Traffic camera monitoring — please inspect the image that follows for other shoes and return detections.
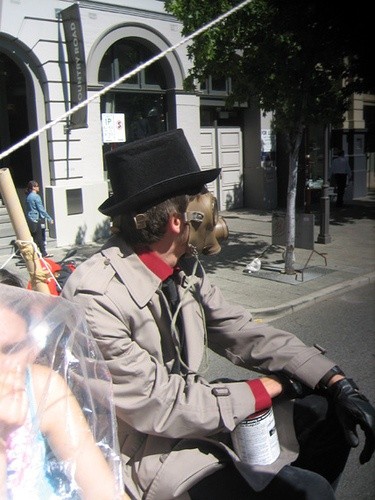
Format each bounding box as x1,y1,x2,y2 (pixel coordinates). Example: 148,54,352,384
45,254,53,257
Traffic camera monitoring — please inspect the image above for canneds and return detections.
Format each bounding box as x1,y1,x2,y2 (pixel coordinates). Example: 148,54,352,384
230,405,280,465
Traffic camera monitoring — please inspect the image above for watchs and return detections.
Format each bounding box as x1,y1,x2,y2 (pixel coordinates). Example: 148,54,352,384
319,365,345,386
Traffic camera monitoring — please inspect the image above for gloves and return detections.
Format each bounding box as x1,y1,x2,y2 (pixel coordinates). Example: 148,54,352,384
274,370,315,399
328,378,375,465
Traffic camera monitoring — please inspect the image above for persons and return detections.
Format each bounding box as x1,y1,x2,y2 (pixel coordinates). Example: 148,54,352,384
47,128,375,500
24,180,54,258
331,150,352,208
0,268,131,499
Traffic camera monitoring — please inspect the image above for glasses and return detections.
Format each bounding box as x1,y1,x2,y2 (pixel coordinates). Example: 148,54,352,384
1,339,29,354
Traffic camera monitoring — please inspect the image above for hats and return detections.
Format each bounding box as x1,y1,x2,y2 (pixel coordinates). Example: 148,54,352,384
98,128,222,218
